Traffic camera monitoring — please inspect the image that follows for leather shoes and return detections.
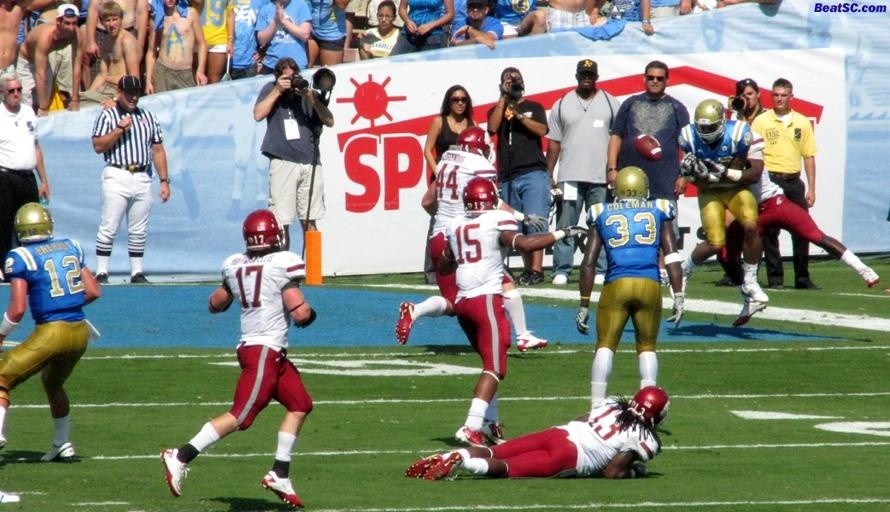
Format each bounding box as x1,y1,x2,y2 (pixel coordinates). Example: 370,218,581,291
95,273,108,283
770,284,784,289
794,278,822,289
130,272,151,283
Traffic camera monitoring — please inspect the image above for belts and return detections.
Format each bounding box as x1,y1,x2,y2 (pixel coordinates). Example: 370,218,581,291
113,164,146,172
769,173,800,180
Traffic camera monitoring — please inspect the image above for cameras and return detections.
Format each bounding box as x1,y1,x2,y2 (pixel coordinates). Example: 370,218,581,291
283,74,312,92
733,97,746,112
507,76,525,92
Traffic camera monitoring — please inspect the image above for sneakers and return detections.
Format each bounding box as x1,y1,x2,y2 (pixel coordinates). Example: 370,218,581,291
664,262,689,298
161,446,191,497
515,270,606,287
732,298,767,327
261,470,306,508
405,418,507,478
516,333,548,352
40,439,77,462
859,267,880,287
741,282,769,303
715,277,735,287
395,301,415,344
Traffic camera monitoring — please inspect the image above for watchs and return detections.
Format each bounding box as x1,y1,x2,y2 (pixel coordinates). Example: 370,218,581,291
159,178,170,182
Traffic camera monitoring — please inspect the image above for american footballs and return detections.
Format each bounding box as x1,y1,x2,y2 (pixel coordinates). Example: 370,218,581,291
634,134,663,160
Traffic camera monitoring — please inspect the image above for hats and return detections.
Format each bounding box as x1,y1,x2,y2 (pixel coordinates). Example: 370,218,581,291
577,59,598,76
467,0,488,6
118,76,142,92
56,3,80,17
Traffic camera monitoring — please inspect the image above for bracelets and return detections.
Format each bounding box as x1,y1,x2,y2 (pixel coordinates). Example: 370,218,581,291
608,167,618,170
116,124,125,129
579,296,589,300
0,312,16,336
553,230,566,240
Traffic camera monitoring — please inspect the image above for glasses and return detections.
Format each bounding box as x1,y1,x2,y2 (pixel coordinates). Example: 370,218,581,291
466,5,484,12
645,75,664,82
449,96,468,104
377,14,393,21
7,86,22,94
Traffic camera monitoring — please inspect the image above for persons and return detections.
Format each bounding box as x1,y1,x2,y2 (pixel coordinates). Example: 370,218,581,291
1,202,100,460
0,75,50,277
395,128,548,353
576,166,684,415
162,209,317,508
253,57,333,254
2,0,353,120
360,0,880,324
438,179,589,447
92,76,171,284
405,385,669,480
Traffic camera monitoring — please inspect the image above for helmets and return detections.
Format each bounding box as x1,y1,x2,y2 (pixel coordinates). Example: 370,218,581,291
456,126,491,159
241,211,281,254
462,177,499,214
615,165,650,200
694,99,725,127
626,386,670,426
13,201,53,244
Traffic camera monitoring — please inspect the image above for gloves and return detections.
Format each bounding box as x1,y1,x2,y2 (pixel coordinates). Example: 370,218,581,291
678,152,728,184
521,212,548,233
575,306,591,335
626,461,649,479
559,224,589,239
666,292,685,330
